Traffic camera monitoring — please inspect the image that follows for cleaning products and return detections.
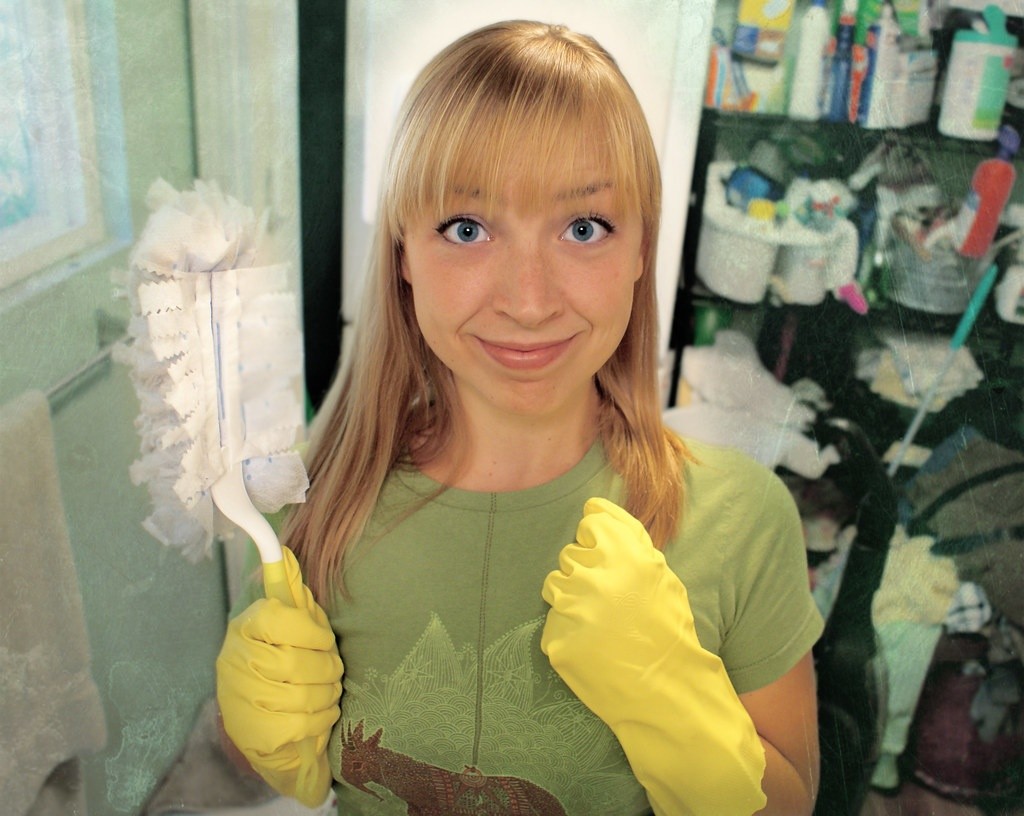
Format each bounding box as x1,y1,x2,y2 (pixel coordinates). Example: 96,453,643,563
789,0,837,120
823,0,900,130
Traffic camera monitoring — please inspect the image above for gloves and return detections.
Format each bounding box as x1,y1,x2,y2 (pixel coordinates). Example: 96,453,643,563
215,546,344,808
540,499,769,814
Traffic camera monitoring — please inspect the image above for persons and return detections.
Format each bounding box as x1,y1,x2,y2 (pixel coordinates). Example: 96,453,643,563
216,19,827,816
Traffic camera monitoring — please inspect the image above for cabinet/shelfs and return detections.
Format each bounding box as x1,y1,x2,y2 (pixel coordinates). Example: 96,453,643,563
658,0,1023,470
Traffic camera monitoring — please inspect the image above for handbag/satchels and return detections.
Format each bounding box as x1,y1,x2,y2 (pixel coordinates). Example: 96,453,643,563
895,423,1024,631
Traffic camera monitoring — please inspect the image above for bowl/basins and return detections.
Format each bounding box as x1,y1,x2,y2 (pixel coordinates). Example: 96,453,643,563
882,243,973,314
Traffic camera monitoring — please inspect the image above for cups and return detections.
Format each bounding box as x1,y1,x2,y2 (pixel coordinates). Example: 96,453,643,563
937,4,1020,143
